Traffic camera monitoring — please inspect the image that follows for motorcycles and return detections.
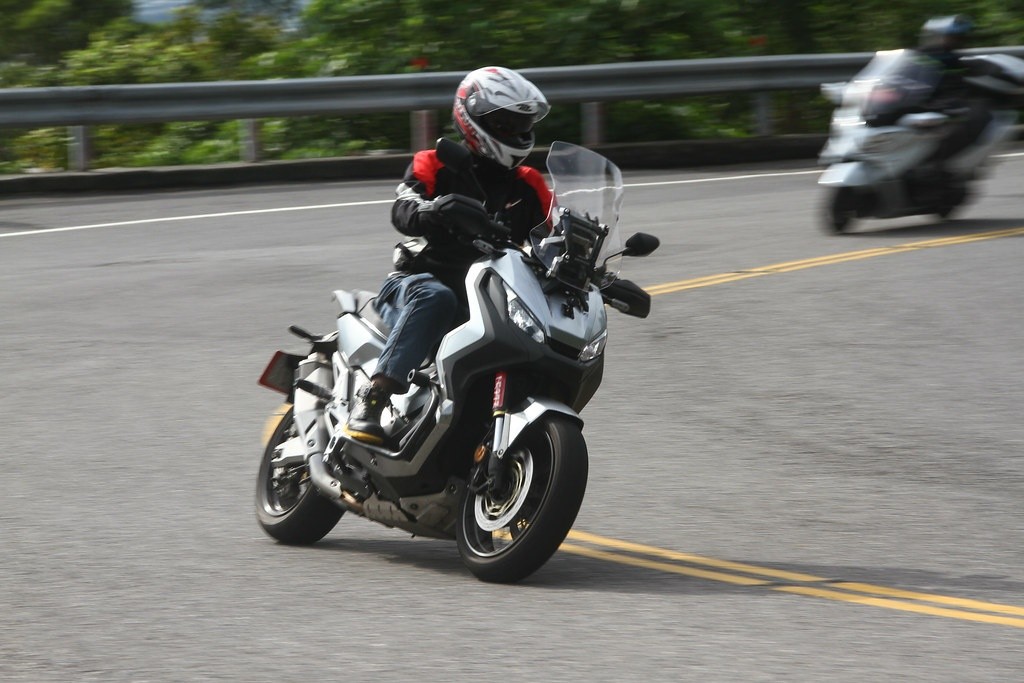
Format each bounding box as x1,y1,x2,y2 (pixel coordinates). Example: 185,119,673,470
254,142,664,588
808,46,1023,237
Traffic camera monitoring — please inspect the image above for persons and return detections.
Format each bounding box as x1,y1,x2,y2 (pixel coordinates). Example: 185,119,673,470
342,66,571,444
869,13,993,214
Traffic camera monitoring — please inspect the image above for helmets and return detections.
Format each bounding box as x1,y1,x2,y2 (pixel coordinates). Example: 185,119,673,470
453,66,550,169
924,15,967,49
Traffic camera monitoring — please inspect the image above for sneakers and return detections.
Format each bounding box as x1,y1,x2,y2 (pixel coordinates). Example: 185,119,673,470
343,387,390,444
